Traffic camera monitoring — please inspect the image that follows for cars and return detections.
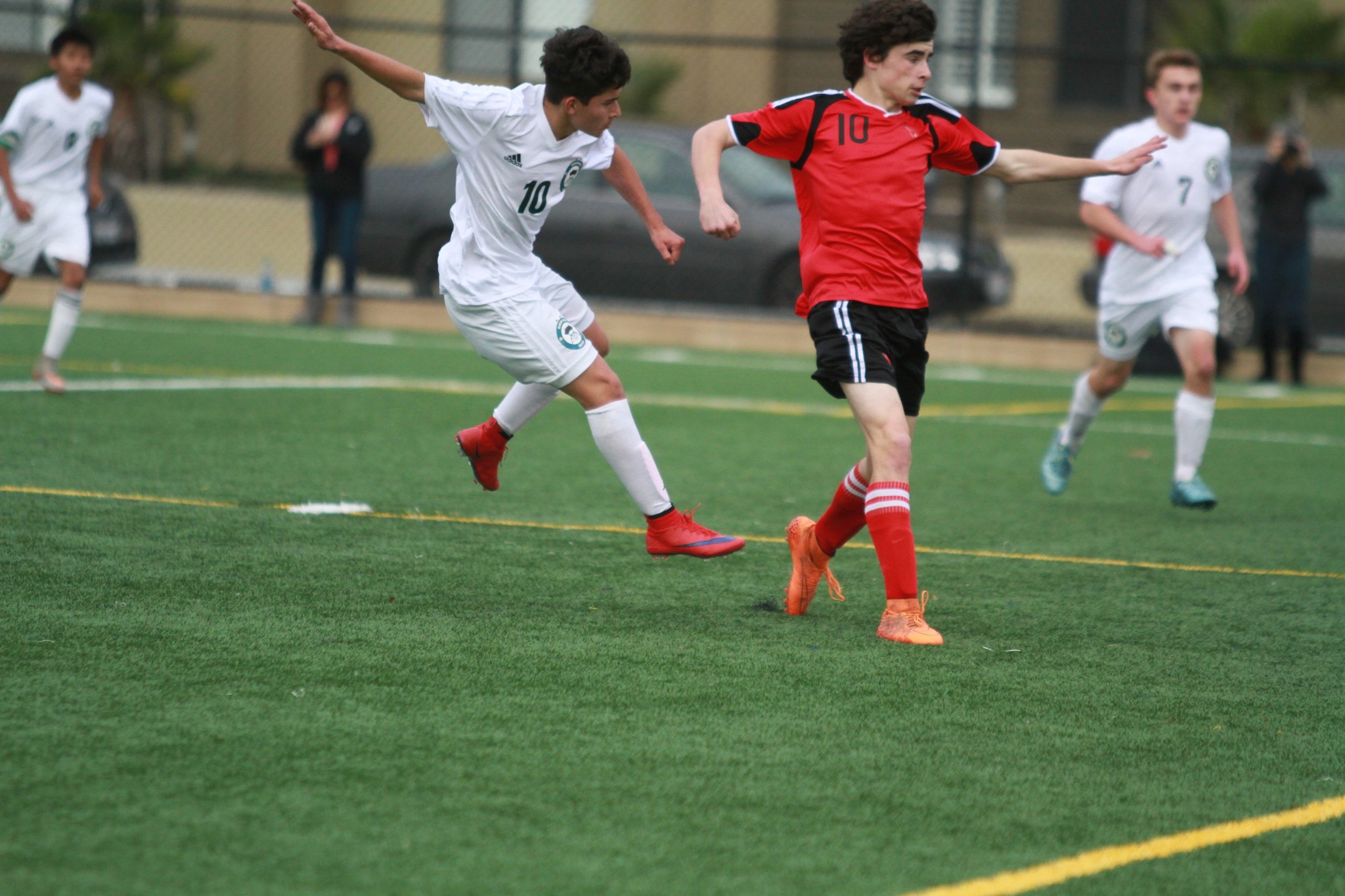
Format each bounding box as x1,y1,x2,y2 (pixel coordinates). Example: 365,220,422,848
31,174,139,280
353,117,1015,322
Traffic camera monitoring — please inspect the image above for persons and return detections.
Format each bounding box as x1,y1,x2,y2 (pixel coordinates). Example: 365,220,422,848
1039,48,1252,509
690,0,1168,646
289,0,747,560
1248,122,1329,386
0,27,114,393
289,66,373,328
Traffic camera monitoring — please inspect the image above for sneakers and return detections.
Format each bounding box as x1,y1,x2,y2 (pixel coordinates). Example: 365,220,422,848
453,414,514,492
31,351,66,393
643,502,746,562
1039,425,1082,496
784,516,845,616
876,591,944,645
1169,472,1218,509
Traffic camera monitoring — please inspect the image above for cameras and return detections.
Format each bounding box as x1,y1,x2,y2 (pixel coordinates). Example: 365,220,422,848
1283,145,1298,156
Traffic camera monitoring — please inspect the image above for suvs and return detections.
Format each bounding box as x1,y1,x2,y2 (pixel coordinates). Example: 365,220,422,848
1074,142,1345,380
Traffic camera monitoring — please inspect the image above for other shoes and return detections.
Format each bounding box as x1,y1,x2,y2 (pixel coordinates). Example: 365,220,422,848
335,291,357,326
292,291,326,325
